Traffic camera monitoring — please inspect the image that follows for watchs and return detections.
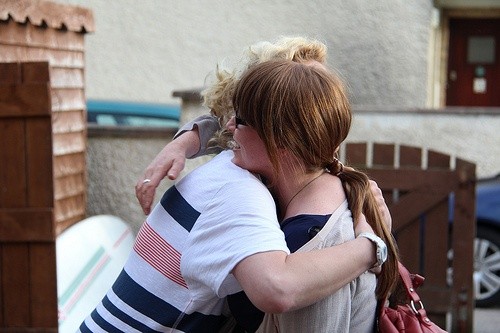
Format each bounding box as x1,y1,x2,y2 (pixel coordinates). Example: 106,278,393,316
355,232,387,268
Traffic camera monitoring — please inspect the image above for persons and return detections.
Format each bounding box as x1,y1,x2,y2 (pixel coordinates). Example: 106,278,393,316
72,35,394,333
134,61,387,333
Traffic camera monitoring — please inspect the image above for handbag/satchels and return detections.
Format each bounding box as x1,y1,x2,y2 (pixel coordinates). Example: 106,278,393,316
378,261,448,333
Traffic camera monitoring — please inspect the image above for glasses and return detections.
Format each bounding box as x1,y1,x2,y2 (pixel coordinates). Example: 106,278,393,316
236,111,249,129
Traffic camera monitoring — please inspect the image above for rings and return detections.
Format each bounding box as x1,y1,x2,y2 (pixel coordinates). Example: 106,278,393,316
142,179,150,183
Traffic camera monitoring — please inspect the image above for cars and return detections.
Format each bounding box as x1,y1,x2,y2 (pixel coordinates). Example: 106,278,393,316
391,172,500,306
85,98,181,128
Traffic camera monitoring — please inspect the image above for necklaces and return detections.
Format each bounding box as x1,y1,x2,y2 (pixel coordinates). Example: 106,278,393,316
284,169,327,221
